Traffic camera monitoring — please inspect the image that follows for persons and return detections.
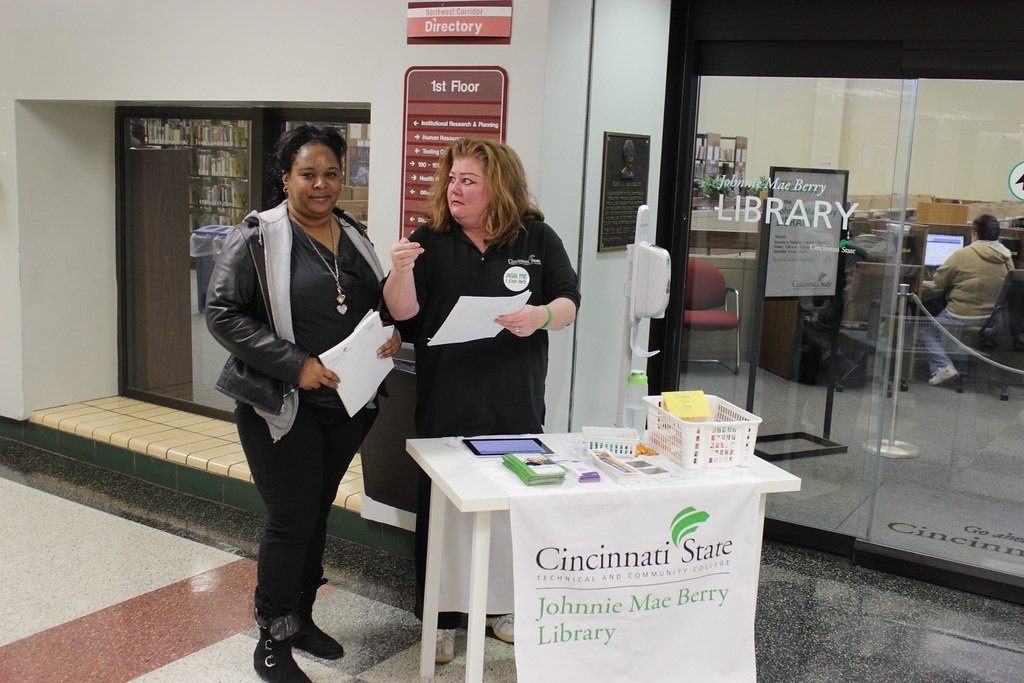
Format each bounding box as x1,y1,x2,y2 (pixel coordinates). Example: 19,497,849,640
801,217,903,386
737,170,743,187
204,123,402,683
378,138,580,662
919,214,1016,386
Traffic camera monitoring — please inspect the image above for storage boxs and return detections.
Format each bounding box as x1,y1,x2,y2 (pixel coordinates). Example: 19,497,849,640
641,393,764,470
917,202,968,225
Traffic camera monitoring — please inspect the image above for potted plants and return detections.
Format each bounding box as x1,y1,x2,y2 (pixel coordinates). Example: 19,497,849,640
696,173,732,199
756,177,768,200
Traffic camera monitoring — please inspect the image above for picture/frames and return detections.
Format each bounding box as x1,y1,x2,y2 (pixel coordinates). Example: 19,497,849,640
597,131,651,251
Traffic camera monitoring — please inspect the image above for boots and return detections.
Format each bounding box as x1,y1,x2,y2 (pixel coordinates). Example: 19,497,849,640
292,566,344,660
254,585,312,682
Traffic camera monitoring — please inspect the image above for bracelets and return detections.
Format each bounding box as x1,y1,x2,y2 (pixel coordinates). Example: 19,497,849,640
540,305,553,329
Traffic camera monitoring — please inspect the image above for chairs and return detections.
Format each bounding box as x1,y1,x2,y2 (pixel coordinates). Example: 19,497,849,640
814,261,923,397
937,268,1024,401
682,262,741,374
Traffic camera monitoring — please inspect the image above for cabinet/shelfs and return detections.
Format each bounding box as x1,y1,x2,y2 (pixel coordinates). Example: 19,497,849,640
130,118,247,267
693,133,747,195
680,210,759,358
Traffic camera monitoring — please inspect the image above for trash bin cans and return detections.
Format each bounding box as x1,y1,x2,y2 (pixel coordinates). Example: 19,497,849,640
359,344,421,514
192,225,235,314
794,297,843,386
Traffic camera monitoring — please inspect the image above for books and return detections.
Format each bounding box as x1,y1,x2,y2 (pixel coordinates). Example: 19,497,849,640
189,206,249,236
197,146,249,177
695,145,706,159
719,148,733,161
190,177,250,207
142,118,249,148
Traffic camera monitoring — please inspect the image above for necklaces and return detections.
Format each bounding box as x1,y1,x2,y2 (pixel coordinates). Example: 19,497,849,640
288,212,348,316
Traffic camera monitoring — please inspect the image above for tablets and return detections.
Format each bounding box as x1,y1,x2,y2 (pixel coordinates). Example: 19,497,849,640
462,437,557,457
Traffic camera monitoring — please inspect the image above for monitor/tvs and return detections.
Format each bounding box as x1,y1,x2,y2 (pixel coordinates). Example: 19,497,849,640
1002,237,1021,265
924,233,966,267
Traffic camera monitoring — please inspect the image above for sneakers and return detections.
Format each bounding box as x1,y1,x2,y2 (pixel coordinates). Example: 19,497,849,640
929,365,957,385
435,629,456,662
486,615,513,641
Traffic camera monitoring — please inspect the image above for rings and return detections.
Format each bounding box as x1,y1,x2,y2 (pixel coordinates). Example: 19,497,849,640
516,327,520,333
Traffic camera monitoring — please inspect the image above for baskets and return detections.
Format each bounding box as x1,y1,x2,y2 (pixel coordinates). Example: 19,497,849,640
643,395,763,469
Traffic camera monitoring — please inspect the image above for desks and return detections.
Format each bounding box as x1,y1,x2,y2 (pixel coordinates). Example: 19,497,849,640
405,437,802,683
